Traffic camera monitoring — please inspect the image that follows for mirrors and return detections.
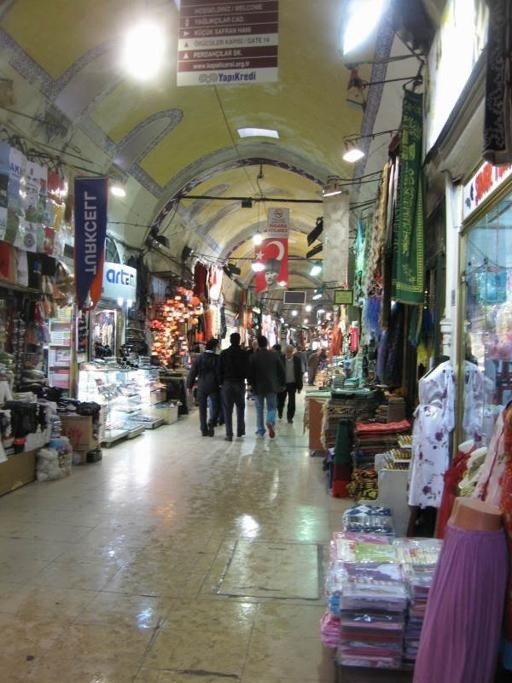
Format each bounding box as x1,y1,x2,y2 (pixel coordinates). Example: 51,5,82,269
453,173,512,457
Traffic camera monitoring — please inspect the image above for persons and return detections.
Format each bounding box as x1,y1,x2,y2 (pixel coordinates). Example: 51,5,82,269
257,258,287,304
213,338,227,428
277,343,302,424
241,334,328,403
214,331,249,443
184,336,222,438
245,334,287,439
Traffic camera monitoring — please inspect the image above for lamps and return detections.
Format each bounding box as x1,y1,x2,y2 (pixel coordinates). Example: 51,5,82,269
342,129,403,163
59,162,127,198
226,201,344,302
322,171,384,198
346,70,423,105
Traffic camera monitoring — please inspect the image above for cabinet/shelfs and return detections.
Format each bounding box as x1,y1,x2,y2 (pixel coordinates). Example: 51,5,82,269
78,366,168,448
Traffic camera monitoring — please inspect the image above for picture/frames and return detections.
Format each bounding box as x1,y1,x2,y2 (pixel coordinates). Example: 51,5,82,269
461,158,512,225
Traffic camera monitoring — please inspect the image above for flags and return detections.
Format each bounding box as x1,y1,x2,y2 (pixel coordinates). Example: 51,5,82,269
254,237,288,304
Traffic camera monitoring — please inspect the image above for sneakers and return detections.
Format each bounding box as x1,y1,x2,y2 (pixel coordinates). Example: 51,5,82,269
277,409,283,420
255,431,264,438
286,418,294,424
224,435,232,441
308,381,317,386
237,432,243,437
266,422,275,439
202,419,224,437
